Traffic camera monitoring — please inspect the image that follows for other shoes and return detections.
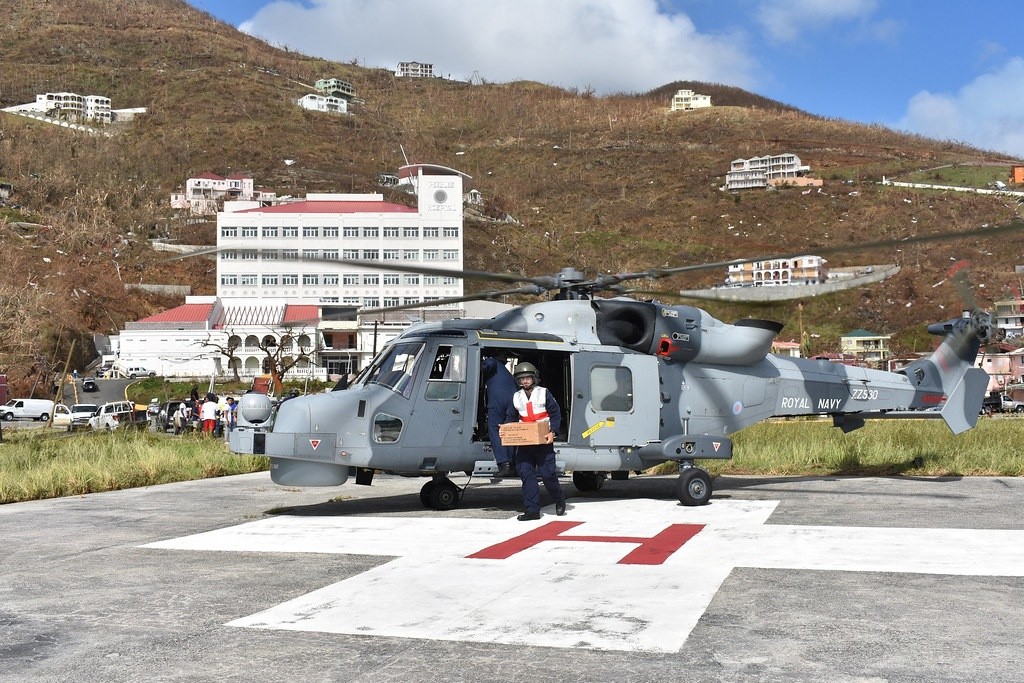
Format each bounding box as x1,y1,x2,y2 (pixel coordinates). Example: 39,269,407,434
493,462,512,476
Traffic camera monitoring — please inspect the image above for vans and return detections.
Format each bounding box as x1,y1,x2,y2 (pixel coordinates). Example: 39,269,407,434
99,364,112,377
0,399,54,423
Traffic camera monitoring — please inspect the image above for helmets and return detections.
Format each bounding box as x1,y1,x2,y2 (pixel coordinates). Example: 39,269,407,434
513,362,541,390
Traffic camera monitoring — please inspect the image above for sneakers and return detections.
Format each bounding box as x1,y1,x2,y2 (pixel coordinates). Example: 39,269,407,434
517,511,540,521
556,501,566,516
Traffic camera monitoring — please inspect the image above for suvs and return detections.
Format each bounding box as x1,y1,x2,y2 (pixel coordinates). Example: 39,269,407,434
126,366,157,381
157,399,190,433
53,403,98,433
149,398,160,415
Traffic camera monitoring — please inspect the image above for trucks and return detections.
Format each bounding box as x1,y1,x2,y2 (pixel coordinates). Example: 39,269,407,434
88,400,148,435
980,392,1024,415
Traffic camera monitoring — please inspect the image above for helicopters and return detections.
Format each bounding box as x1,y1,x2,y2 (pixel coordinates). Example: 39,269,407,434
159,220,1024,514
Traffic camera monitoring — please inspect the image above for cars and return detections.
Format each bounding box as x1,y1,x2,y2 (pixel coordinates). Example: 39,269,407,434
81,376,95,387
83,381,96,393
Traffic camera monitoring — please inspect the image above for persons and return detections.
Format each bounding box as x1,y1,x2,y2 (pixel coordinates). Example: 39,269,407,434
172,392,238,440
497,361,565,521
479,356,520,479
275,387,299,412
73,368,78,378
191,384,199,402
